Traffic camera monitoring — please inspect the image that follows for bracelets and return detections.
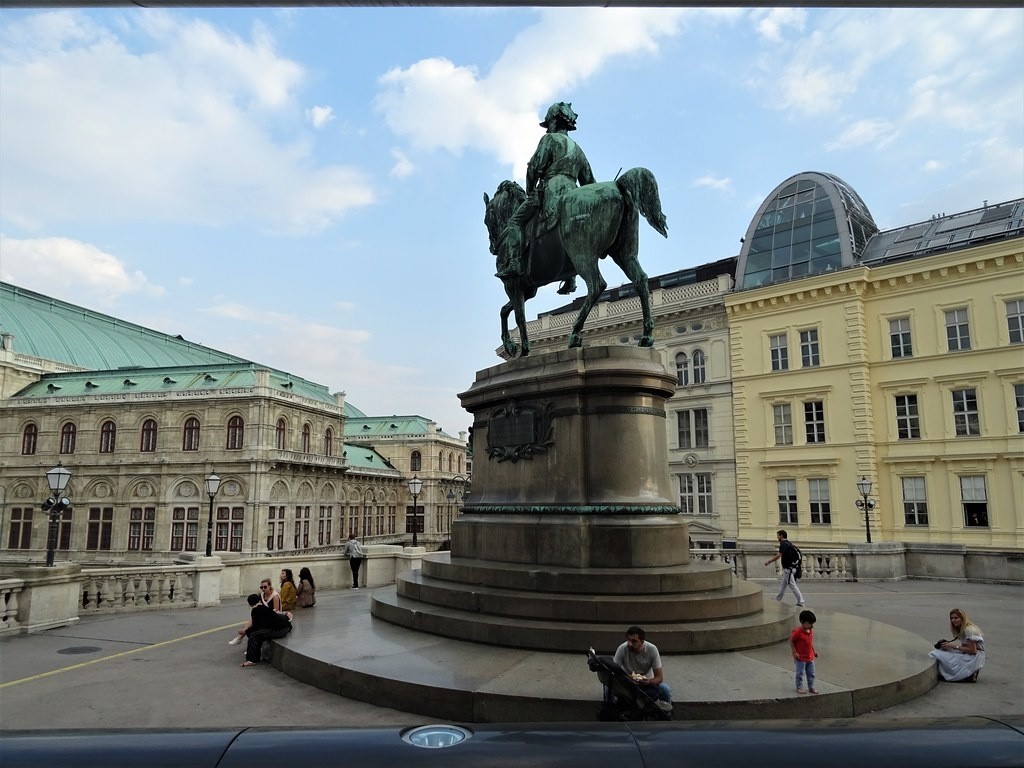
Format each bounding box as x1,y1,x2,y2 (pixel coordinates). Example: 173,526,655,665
956,645,958,649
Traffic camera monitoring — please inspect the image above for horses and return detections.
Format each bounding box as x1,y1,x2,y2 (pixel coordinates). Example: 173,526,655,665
483,166,669,359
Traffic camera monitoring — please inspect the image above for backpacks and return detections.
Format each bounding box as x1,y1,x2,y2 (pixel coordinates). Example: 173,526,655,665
350,541,363,558
786,541,802,568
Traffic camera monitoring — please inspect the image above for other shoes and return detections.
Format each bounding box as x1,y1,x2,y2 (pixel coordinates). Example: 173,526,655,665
350,586,358,590
240,661,258,667
795,601,804,607
809,688,818,694
796,688,807,694
971,669,979,683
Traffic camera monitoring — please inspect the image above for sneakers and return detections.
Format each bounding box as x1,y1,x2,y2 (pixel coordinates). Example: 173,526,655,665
228,638,242,646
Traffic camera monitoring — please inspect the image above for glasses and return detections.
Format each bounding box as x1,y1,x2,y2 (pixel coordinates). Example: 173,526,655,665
260,586,268,590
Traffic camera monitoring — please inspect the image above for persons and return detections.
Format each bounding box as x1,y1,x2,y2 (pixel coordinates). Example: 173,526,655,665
228,578,280,646
790,609,818,694
343,535,363,590
237,593,287,666
495,101,596,292
602,627,671,721
764,530,805,607
295,567,317,607
928,608,985,682
278,568,298,610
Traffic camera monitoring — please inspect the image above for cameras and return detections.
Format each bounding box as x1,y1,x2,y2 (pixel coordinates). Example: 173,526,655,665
934,639,947,651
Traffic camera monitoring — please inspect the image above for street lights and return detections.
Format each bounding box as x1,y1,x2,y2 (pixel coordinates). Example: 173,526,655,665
854,475,876,544
406,474,423,547
204,468,223,557
41,460,73,566
